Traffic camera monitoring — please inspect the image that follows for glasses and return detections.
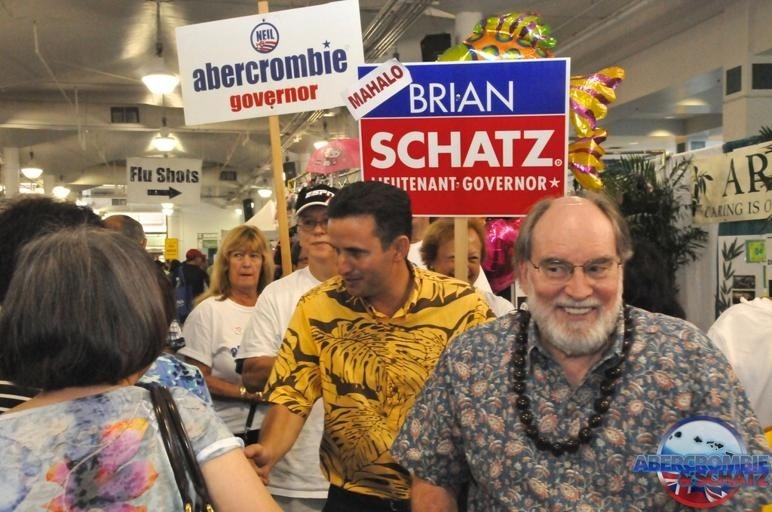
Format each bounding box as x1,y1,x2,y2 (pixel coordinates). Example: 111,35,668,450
527,259,620,279
297,217,329,232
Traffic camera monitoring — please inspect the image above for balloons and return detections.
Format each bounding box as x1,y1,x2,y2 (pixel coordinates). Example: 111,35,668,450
433,13,626,137
568,129,608,195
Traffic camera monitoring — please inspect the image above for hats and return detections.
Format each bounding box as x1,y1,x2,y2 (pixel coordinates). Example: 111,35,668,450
295,184,338,216
186,248,208,257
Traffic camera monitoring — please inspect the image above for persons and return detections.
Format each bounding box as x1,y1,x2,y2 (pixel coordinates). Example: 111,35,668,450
0,179,771,511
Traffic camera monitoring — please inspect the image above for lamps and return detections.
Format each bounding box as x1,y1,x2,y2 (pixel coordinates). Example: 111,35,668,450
235,123,330,224
142,0,180,97
53,174,99,214
20,130,43,179
154,97,176,152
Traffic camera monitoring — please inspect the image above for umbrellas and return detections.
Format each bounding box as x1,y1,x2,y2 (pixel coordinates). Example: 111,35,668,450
303,137,361,174
